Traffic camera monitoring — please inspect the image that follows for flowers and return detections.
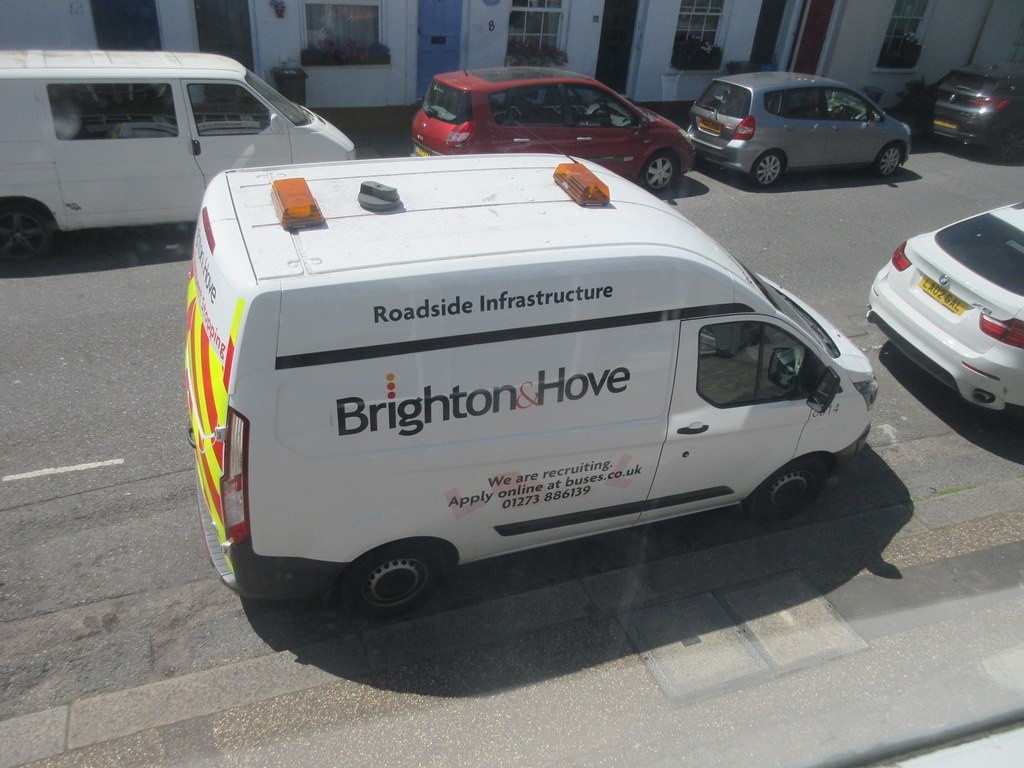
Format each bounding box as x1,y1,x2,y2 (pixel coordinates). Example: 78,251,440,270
301,37,389,62
270,0,284,9
506,39,568,68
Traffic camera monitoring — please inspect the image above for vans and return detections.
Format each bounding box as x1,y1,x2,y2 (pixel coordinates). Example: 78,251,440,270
0,49,358,269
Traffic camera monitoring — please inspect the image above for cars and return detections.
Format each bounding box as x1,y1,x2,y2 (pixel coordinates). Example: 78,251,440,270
863,201,1024,417
911,60,1024,166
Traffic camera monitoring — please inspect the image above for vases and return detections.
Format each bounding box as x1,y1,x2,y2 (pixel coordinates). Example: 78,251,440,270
670,36,720,66
276,8,284,18
684,54,721,69
303,56,390,65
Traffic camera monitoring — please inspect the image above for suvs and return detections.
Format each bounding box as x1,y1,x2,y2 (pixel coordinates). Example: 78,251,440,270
686,70,913,190
409,64,696,195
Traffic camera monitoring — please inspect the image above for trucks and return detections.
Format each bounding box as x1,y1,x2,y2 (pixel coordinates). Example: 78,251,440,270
183,153,880,624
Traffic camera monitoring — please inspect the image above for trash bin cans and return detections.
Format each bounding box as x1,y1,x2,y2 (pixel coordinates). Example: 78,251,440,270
272,68,310,106
726,61,762,76
